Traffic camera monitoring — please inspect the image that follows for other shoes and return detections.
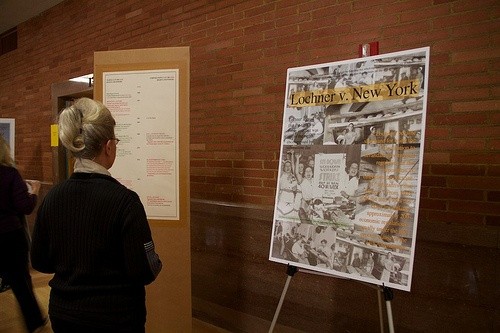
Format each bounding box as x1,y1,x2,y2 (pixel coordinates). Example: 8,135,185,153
31,318,48,333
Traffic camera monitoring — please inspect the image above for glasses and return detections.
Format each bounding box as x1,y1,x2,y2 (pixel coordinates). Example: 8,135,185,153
105,137,120,145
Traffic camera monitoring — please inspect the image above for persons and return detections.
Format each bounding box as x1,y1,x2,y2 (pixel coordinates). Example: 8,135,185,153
272,54,424,282
0,134,50,333
30,97,163,333
